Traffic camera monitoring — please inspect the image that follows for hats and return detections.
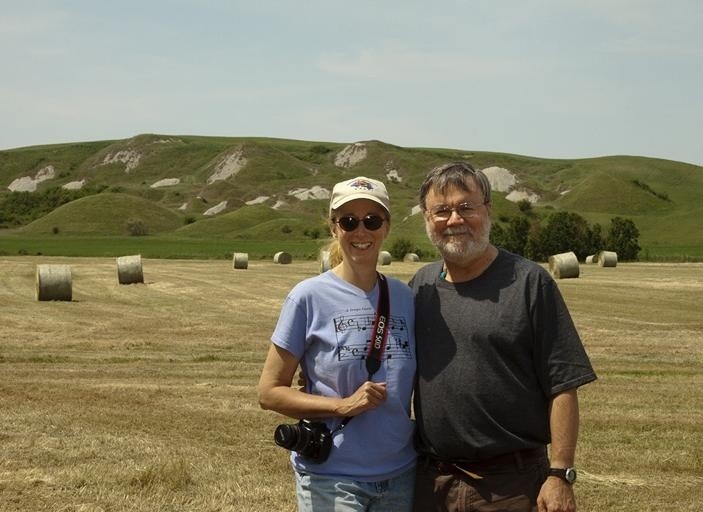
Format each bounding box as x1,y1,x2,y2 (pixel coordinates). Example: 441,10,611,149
329,176,392,220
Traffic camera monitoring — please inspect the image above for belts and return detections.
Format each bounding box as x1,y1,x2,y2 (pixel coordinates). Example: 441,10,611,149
423,445,546,480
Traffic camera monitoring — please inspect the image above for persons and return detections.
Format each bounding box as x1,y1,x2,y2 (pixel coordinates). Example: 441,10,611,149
257,177,420,512
298,163,598,512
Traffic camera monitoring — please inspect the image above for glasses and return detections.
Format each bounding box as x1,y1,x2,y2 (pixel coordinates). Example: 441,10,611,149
334,213,387,230
425,201,484,221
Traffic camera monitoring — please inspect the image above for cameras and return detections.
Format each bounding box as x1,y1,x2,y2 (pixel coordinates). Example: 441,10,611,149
274,419,332,464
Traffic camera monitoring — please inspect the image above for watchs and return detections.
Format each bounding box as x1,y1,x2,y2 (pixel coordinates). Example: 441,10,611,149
547,467,577,485
